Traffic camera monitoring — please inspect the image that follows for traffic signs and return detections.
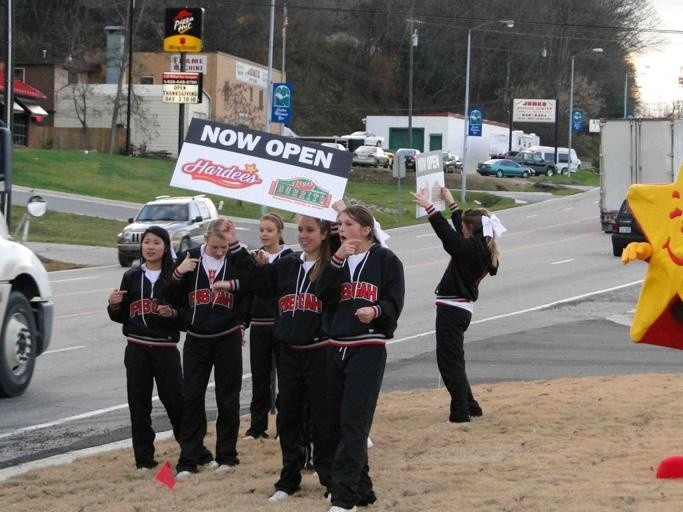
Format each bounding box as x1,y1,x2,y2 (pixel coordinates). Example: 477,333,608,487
161,71,199,104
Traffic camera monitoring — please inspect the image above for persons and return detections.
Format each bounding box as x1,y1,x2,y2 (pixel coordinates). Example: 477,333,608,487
212,216,332,499
323,216,349,285
409,186,506,423
154,215,256,479
107,226,218,474
244,215,301,440
315,206,405,511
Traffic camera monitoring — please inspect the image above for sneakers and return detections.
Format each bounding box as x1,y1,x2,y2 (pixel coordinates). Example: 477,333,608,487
268,490,304,503
367,436,374,449
137,467,147,472
321,492,332,501
242,435,254,441
197,461,219,471
176,471,190,479
328,506,356,512
216,465,234,474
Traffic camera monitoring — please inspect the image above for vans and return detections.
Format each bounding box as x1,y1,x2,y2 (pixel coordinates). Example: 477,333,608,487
0,121,54,397
523,146,577,175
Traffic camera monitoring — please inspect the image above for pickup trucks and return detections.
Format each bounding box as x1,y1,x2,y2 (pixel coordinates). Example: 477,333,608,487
490,151,555,177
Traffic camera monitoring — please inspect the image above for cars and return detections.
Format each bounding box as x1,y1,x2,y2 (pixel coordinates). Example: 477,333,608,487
475,158,531,178
347,130,461,176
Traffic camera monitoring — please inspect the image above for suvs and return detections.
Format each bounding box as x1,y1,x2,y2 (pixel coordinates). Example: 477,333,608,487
116,194,219,268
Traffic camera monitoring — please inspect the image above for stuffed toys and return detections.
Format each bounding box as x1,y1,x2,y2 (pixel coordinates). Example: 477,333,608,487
620,160,683,478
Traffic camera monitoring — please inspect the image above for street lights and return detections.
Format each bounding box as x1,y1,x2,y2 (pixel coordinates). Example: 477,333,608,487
567,46,603,178
460,19,514,206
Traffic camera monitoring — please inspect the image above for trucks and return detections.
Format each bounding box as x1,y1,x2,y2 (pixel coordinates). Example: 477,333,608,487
599,118,682,256
